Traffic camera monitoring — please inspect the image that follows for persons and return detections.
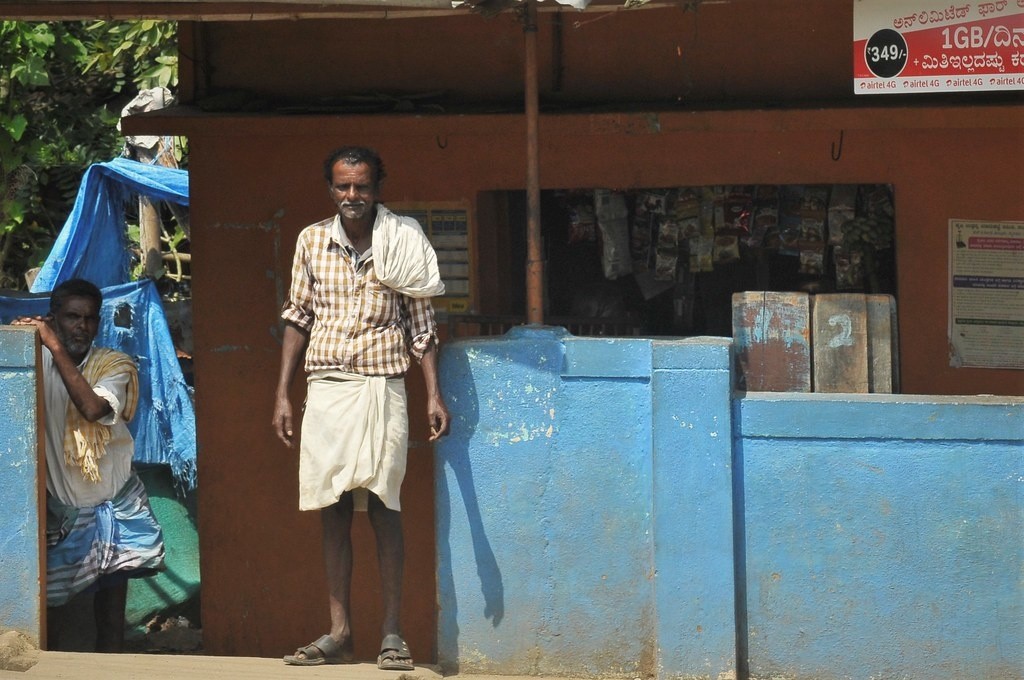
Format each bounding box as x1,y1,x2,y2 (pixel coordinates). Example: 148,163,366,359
271,145,450,670
10,279,165,653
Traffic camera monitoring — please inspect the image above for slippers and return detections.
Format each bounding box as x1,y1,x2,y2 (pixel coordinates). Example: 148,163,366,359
283,634,355,665
377,635,414,669
